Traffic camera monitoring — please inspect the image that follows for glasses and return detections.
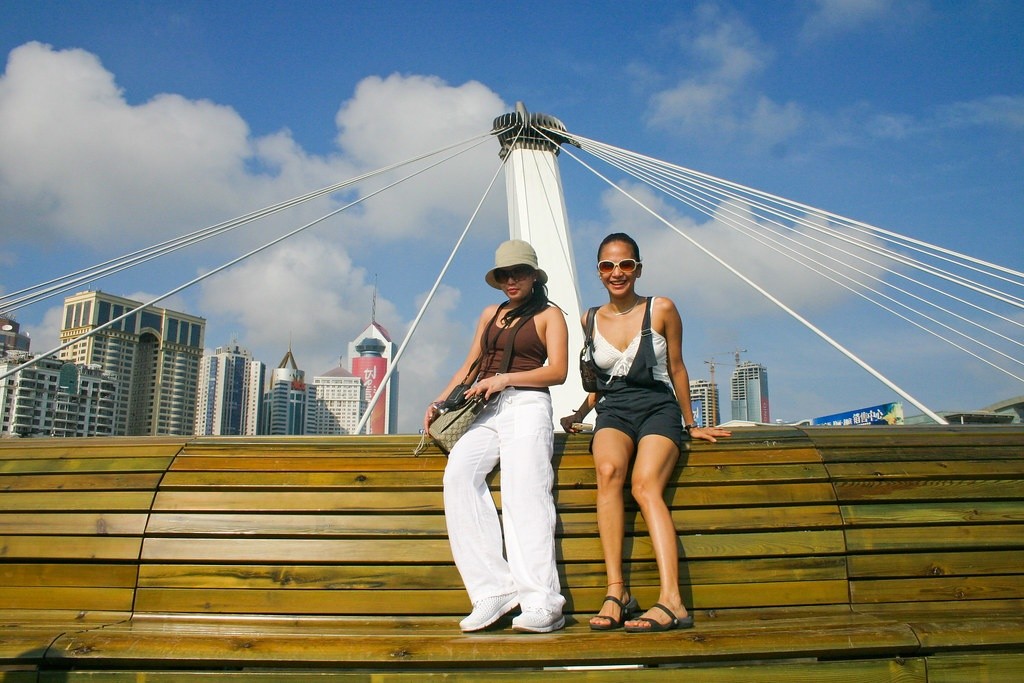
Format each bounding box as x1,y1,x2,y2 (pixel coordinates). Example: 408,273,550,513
494,266,534,283
597,259,641,274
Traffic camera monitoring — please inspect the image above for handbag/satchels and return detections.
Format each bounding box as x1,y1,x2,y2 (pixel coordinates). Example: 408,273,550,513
580,307,598,392
428,395,488,455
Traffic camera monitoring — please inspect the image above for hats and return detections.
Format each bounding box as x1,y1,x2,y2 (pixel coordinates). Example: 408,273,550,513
485,240,548,290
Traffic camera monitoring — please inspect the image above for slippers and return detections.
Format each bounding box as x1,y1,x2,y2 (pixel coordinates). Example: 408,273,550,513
590,596,638,630
624,604,694,633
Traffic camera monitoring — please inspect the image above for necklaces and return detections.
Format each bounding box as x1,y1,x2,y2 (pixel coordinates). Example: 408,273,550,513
612,295,640,316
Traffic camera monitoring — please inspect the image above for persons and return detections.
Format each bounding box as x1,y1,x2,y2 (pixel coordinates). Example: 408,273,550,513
559,232,731,632
424,239,568,634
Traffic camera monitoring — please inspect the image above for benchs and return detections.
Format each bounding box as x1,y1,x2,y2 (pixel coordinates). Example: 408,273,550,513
0,427,1024,683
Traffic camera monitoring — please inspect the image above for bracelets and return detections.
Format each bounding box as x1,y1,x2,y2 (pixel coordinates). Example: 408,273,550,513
685,421,699,430
572,408,584,422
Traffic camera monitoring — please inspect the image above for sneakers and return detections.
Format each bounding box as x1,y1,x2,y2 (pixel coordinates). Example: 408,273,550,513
511,608,565,633
459,590,520,633
